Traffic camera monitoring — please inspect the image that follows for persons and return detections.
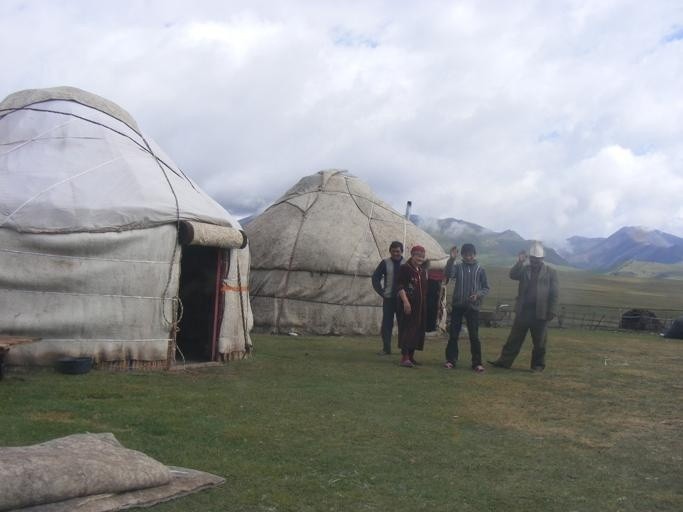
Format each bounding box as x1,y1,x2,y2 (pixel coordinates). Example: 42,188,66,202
395,245,429,368
442,243,490,373
486,239,560,373
371,240,407,356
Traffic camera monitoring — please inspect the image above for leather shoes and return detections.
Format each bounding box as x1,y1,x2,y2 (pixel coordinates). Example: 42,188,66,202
487,360,505,368
376,350,388,356
529,367,543,373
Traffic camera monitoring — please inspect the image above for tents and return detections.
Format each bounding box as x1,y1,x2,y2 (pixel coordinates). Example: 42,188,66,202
0,84,255,374
242,167,449,335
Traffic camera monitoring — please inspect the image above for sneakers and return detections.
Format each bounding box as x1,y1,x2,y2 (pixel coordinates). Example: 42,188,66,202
443,362,454,369
474,365,485,372
399,360,414,367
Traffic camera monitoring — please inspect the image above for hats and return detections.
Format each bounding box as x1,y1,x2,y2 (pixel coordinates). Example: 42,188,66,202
411,246,426,257
529,245,545,257
